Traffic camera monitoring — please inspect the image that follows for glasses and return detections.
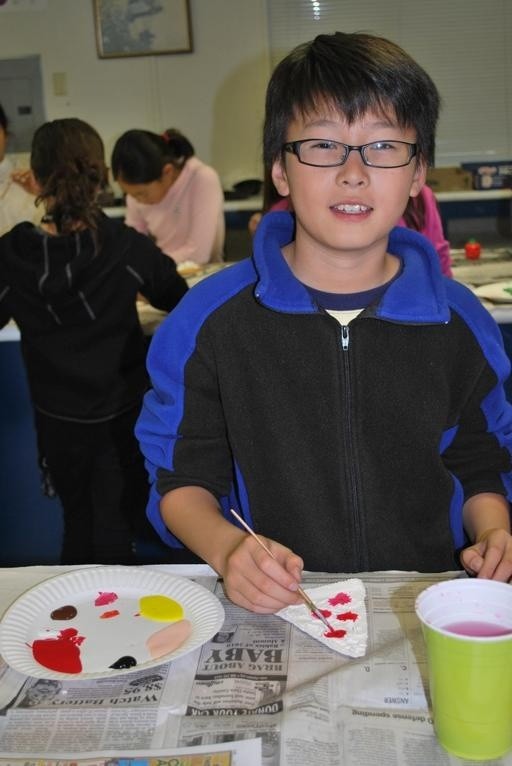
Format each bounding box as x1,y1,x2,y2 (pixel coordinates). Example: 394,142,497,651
280,135,419,170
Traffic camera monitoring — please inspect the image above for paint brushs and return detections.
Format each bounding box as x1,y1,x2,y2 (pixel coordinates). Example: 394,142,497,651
230,508,336,635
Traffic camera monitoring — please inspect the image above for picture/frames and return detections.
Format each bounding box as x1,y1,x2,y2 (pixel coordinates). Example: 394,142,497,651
92,1,193,59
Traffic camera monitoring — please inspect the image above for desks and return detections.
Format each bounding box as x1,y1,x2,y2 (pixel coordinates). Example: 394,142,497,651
1,187,512,341
1,565,511,765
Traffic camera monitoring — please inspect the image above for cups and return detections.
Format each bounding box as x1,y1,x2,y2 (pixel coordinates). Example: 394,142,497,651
412,576,512,762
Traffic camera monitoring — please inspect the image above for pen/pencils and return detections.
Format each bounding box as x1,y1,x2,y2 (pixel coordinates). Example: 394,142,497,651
196,261,227,277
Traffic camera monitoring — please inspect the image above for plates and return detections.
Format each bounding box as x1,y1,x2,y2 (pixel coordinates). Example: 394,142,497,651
0,562,229,682
475,281,512,304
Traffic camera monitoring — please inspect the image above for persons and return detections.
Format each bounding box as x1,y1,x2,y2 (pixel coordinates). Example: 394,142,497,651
134,25,511,618
109,124,228,267
257,158,456,282
1,104,58,244
0,116,192,565
